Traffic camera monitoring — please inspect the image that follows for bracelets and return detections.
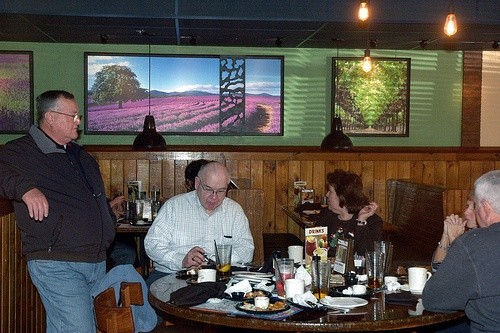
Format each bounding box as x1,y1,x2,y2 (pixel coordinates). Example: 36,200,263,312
437,241,450,252
355,216,367,226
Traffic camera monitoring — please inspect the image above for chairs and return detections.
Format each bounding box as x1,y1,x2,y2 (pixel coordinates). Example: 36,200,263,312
94,282,143,333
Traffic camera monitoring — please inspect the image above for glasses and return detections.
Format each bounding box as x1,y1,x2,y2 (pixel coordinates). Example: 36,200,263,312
50,110,82,123
196,176,228,194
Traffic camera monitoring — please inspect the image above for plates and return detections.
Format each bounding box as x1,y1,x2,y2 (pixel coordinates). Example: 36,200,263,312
223,290,272,302
186,277,198,284
236,297,290,314
320,297,368,310
331,285,374,299
130,220,151,226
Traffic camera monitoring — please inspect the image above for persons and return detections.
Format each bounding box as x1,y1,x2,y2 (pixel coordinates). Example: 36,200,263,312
422,169,500,333
0,89,116,333
430,191,479,275
143,161,256,326
314,170,384,260
108,195,145,267
185,159,212,191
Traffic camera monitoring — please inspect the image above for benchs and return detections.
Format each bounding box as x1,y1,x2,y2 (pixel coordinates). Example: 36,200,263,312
387,179,447,263
228,180,303,262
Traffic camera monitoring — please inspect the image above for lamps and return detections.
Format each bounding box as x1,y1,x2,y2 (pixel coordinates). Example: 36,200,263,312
132,36,167,148
320,41,354,152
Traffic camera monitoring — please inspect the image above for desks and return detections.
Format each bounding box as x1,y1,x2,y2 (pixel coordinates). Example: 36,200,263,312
282,207,398,234
148,271,466,333
115,225,151,262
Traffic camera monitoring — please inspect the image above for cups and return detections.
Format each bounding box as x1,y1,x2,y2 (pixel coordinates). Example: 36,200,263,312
275,258,294,296
366,241,387,289
384,276,398,291
215,244,232,280
123,200,144,222
311,261,331,299
288,245,303,266
407,267,432,295
306,237,328,260
285,279,304,296
254,296,269,308
369,290,386,321
152,185,161,221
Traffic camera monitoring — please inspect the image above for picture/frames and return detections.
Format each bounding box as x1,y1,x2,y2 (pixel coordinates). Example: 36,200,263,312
84,51,284,136
0,50,34,134
332,57,411,137
